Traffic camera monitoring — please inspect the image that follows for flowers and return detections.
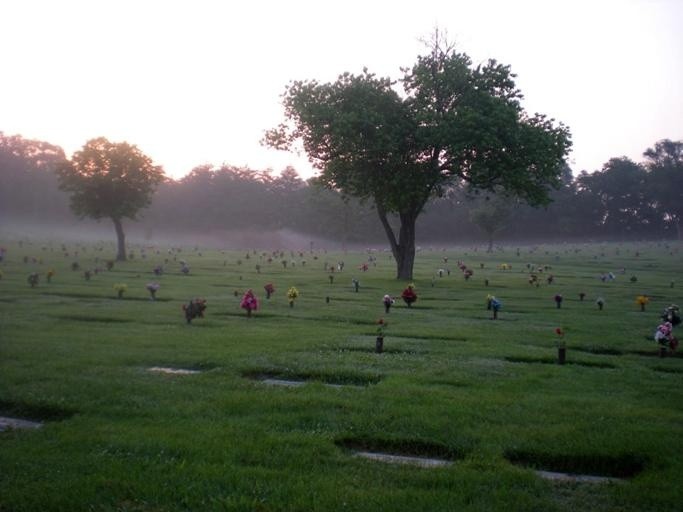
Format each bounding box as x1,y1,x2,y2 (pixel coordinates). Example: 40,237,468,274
182,298,207,323
577,292,586,301
239,288,258,316
27,260,681,287
287,287,299,307
553,293,563,308
659,302,681,326
381,294,395,313
490,299,502,319
145,281,159,300
111,282,128,298
400,287,417,307
595,296,605,310
370,318,388,351
653,320,675,358
636,292,648,310
553,324,570,360
484,293,495,309
263,284,275,299
